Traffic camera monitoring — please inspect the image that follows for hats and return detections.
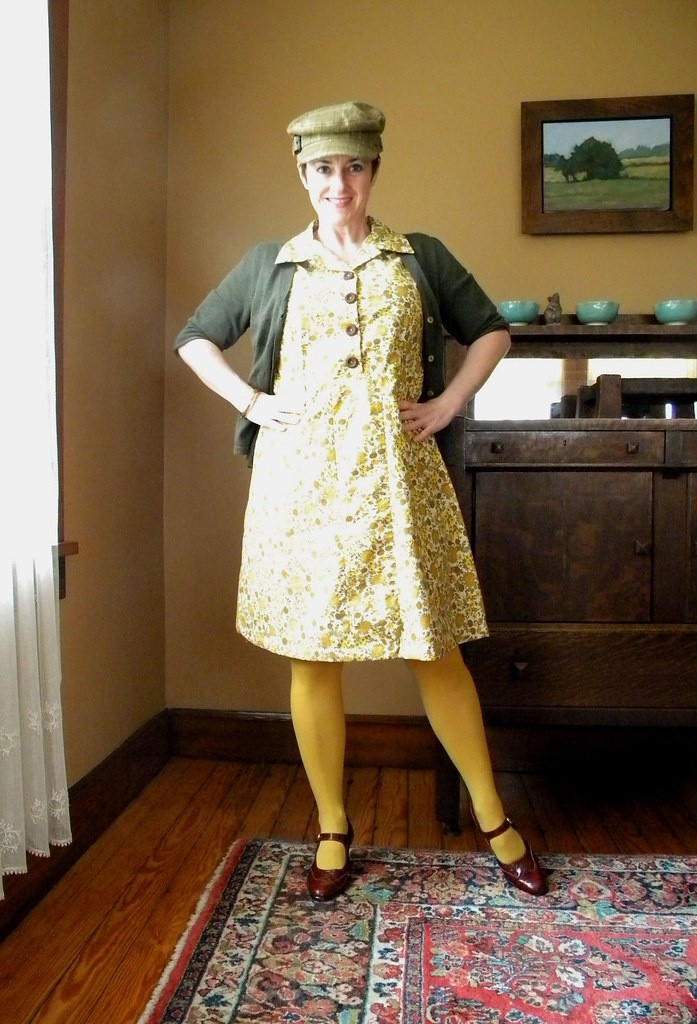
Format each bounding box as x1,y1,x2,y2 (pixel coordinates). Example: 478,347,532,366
287,101,386,164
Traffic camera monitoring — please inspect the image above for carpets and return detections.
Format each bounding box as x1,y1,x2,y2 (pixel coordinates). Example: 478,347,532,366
136,829,697,1024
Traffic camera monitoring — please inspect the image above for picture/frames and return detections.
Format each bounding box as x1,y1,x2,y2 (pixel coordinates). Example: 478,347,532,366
520,94,694,235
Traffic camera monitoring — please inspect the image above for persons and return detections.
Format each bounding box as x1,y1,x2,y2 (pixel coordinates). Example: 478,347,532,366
175,99,548,900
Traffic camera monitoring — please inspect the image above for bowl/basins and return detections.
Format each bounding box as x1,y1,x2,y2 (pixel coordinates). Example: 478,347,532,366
499,302,539,325
653,299,697,325
576,301,620,326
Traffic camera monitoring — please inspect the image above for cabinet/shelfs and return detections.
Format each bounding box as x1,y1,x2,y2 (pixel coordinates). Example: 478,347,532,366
436,313,696,829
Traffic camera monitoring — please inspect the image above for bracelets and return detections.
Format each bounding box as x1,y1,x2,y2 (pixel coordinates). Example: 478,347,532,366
240,390,259,418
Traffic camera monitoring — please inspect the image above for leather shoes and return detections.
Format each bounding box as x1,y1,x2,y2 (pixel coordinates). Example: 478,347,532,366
307,814,354,901
470,797,546,895
